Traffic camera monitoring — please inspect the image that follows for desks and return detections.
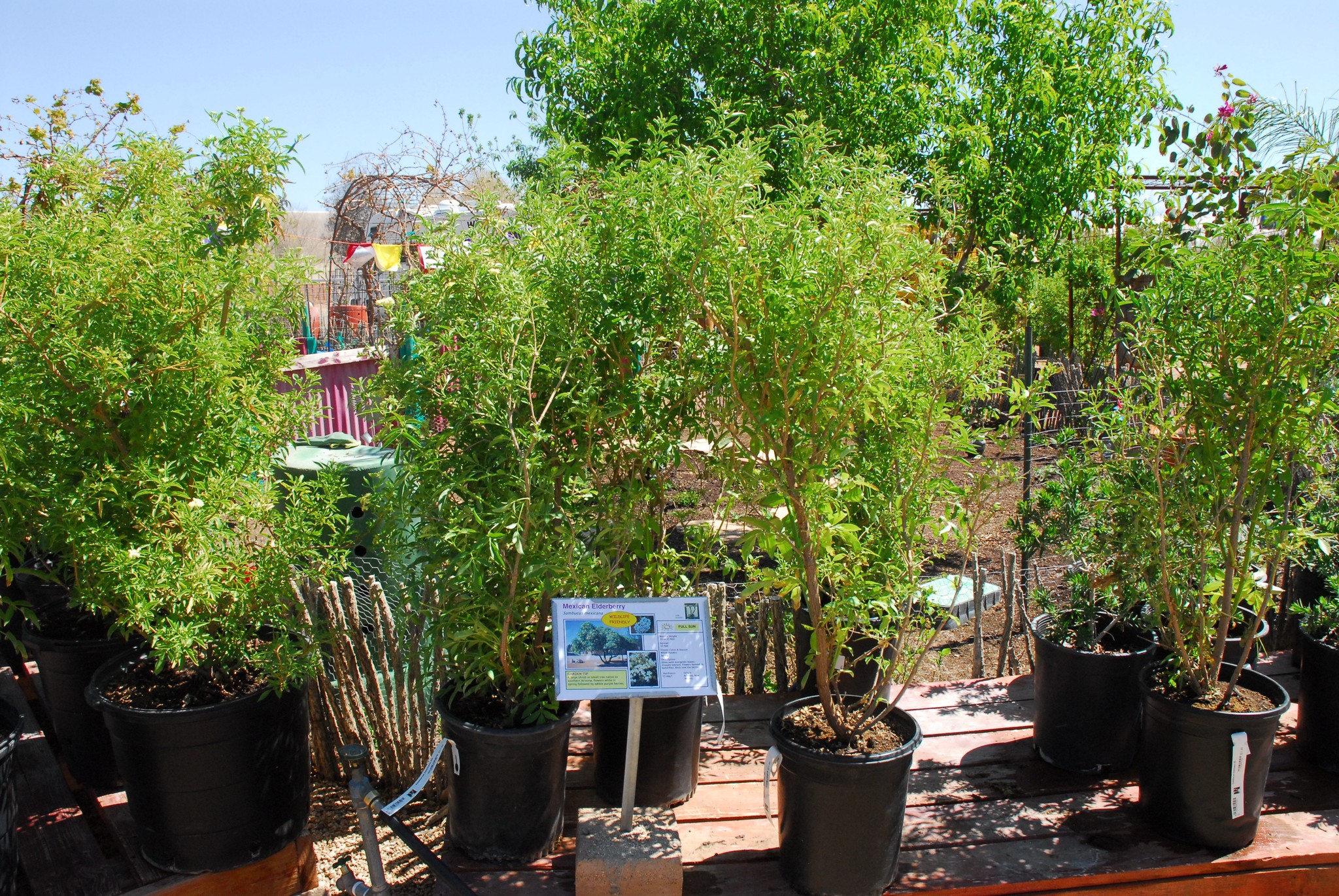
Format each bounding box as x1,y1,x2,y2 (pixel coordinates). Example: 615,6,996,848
438,674,1339,896
0,659,317,896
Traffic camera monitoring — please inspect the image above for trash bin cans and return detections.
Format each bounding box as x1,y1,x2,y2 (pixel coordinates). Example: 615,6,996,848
269,433,442,673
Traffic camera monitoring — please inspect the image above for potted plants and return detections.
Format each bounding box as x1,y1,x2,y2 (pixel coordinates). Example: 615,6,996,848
0,83,1339,896
